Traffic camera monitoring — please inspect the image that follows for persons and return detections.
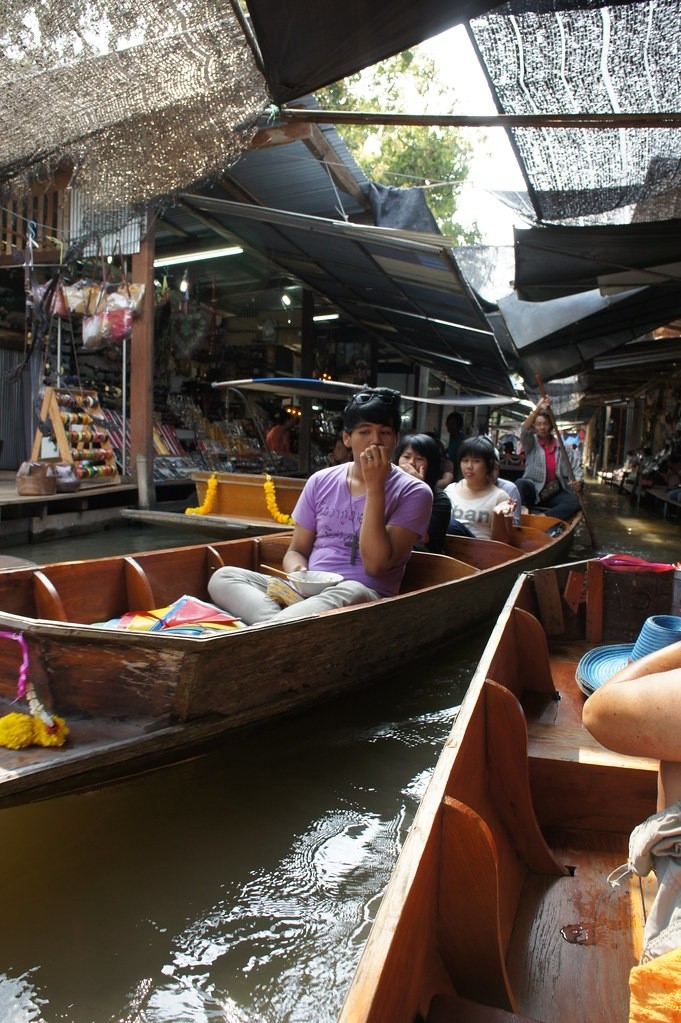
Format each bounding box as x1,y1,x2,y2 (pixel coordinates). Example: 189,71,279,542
582,639,681,1023
513,395,582,520
436,459,454,488
443,435,522,544
394,434,451,554
324,437,354,469
208,389,434,627
519,452,526,467
502,451,511,465
446,411,467,462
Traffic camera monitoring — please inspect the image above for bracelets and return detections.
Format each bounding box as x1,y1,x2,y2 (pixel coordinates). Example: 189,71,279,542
529,411,535,417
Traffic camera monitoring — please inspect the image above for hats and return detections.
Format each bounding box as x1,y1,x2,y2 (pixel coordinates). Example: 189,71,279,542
575,615,681,696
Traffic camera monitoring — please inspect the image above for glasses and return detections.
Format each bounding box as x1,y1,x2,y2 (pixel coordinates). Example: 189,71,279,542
344,392,404,412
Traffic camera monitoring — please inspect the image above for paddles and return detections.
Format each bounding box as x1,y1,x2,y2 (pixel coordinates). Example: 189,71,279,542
534,372,600,548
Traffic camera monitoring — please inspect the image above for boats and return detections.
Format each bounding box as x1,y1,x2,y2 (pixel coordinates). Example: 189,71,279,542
334,552,681,1023
1,497,584,800
118,450,580,544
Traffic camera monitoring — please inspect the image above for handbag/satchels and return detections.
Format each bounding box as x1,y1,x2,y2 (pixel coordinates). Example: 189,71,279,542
37,271,145,348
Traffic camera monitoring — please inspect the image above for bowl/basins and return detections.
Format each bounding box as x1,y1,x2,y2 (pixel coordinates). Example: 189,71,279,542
287,571,344,597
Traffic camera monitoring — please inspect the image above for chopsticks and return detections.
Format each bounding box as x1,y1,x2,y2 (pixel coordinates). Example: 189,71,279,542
261,564,305,582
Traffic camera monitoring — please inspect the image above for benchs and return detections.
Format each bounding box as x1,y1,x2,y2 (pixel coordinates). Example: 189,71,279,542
258,514,570,597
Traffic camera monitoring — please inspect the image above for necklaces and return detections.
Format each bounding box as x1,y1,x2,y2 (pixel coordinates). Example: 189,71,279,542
350,466,361,566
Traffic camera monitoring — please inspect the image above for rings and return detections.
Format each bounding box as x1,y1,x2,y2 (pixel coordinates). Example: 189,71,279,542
367,456,373,460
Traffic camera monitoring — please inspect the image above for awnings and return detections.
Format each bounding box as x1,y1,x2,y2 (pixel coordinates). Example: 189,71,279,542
178,0,681,399
